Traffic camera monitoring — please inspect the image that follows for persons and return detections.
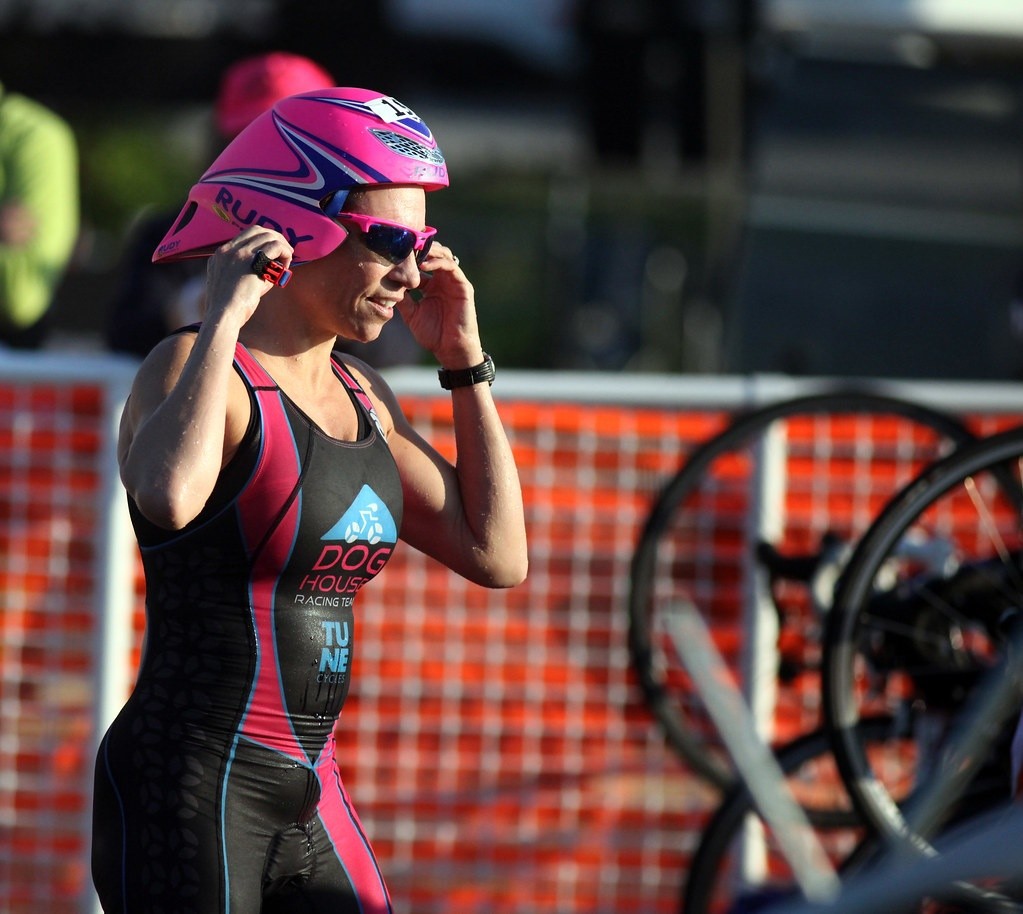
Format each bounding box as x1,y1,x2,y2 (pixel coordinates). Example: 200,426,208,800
0,84,82,353
91,87,532,914
95,50,404,371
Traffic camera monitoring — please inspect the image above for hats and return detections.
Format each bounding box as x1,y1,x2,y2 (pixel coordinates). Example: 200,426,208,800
213,59,336,129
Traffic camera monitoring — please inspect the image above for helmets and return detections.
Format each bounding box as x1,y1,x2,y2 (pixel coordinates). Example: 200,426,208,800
151,89,449,272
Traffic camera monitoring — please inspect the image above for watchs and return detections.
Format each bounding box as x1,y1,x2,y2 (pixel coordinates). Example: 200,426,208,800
436,350,497,391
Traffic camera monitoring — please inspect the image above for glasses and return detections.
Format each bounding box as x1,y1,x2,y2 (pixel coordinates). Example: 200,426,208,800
337,210,438,267
